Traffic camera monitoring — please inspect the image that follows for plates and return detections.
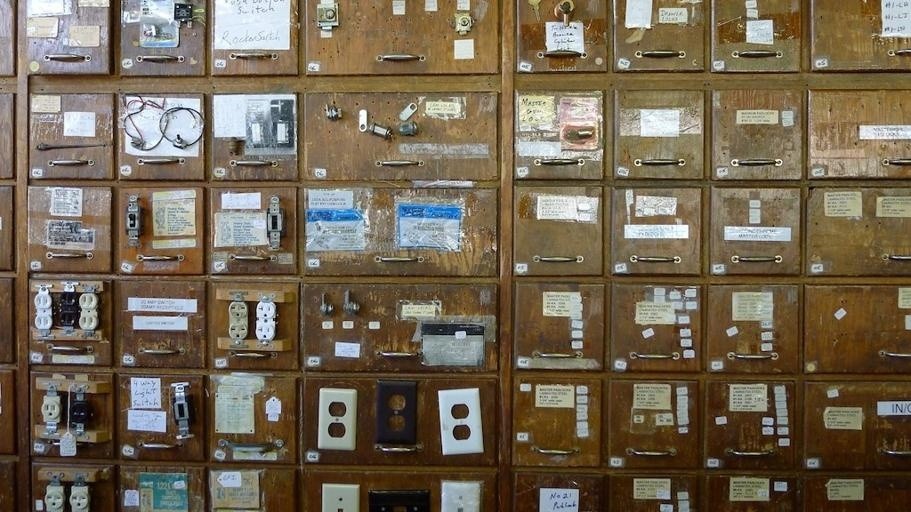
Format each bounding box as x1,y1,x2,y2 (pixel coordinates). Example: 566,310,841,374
0,1,909,512
